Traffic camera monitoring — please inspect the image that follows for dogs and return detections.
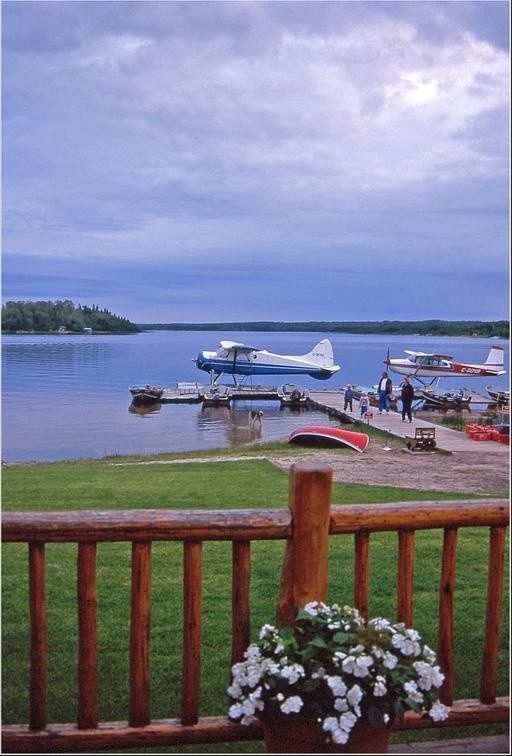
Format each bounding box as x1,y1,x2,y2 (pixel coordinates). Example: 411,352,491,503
248,409,264,428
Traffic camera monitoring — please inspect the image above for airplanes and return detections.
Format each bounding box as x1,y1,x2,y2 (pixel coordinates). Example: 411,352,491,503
484,383,509,404
382,345,506,389
191,338,341,390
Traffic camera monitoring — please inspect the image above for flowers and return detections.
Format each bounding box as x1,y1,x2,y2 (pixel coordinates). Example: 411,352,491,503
225,600,454,750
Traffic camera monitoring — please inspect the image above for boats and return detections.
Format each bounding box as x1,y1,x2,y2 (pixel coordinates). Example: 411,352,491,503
128,384,163,410
276,381,310,407
288,427,369,452
201,386,232,407
421,391,472,406
352,385,397,408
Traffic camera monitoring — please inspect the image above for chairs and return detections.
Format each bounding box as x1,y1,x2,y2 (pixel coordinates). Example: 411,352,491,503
405,427,435,451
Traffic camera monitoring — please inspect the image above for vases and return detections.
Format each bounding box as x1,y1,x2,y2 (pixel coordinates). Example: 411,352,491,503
260,712,389,755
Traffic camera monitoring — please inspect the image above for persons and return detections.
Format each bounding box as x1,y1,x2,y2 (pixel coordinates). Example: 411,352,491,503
356,390,370,419
400,377,414,424
376,370,393,416
343,383,353,412
